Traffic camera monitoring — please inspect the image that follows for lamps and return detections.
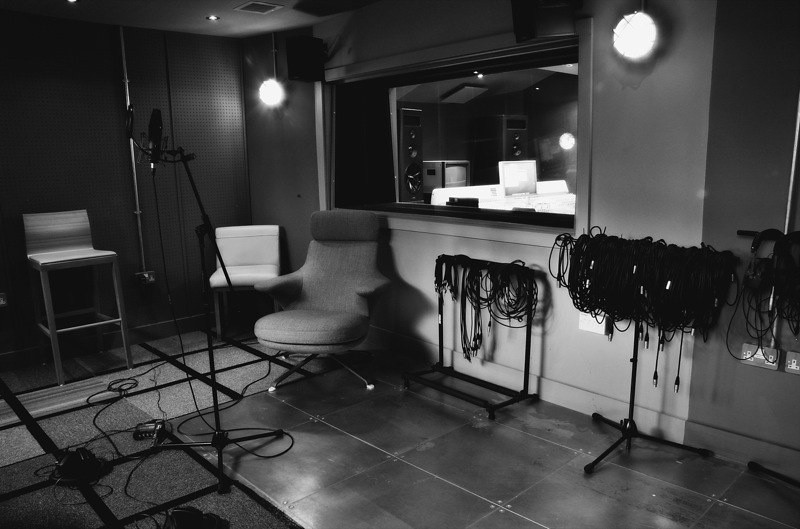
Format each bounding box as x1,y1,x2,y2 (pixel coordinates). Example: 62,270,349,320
611,0,660,63
259,33,287,108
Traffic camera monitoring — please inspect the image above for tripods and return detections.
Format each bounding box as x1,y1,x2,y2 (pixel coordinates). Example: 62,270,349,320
151,147,285,496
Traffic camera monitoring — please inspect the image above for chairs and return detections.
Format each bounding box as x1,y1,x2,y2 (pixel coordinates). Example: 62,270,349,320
209,224,282,341
253,210,393,394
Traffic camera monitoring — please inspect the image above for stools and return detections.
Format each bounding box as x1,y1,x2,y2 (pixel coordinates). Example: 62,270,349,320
21,210,133,387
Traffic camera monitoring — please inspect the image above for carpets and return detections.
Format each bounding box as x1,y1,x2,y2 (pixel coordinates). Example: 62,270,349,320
0,324,353,529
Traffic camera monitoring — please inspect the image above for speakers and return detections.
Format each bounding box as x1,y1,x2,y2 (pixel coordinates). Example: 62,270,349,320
286,35,323,82
511,0,535,43
